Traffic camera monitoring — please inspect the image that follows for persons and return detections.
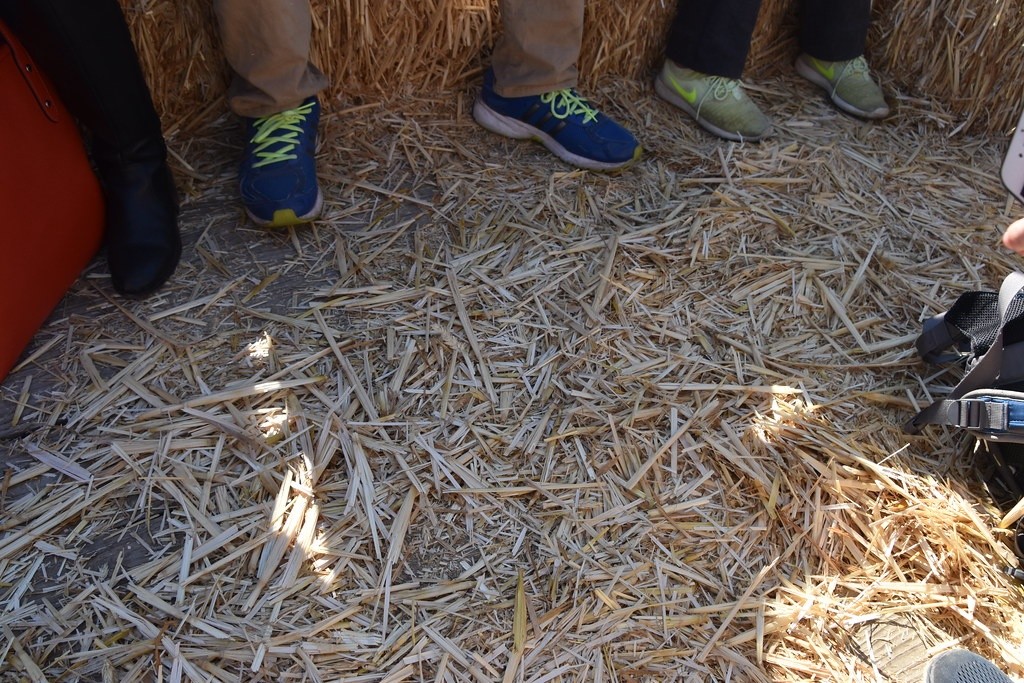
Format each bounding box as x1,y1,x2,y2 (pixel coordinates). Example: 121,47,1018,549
209,0,641,227
0,0,182,298
924,217,1024,683
651,0,891,144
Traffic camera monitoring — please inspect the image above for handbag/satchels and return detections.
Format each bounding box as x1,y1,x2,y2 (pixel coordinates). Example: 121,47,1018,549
0,25,104,387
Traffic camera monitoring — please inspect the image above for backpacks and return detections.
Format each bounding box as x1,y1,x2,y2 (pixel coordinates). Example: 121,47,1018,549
901,271,1024,555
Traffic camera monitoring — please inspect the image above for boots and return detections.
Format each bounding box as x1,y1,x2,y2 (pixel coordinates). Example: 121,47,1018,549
0,0,182,301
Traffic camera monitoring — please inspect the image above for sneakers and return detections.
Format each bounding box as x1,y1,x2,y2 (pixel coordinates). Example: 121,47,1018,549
794,51,889,118
653,58,774,142
929,647,1016,683
473,65,642,174
240,94,323,226
